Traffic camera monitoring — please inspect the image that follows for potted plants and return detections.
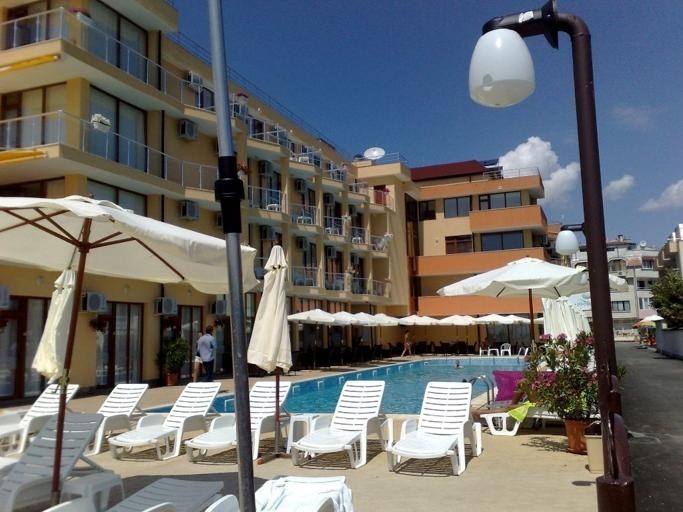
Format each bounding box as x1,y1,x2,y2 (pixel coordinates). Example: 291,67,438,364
154,338,191,386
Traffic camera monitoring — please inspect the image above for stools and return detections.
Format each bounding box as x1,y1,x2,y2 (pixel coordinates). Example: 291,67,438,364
286,412,323,459
59,472,125,509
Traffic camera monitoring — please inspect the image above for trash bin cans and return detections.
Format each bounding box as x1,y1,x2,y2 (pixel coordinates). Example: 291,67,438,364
583,420,605,474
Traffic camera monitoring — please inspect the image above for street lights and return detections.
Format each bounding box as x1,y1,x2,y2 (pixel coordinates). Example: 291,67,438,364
462,1,635,512
552,221,585,257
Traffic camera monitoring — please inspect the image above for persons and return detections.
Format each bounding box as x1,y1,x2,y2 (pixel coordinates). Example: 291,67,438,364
196,324,217,382
482,336,490,350
401,330,412,358
539,327,544,340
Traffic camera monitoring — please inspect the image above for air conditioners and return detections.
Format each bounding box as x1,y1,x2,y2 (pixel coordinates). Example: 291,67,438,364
177,67,360,265
77,290,108,314
209,299,227,316
150,297,178,317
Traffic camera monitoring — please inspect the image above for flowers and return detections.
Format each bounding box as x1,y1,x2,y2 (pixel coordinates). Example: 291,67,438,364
513,330,632,417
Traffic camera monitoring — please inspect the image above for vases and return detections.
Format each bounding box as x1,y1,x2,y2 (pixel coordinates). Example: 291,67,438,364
584,433,606,474
563,413,588,456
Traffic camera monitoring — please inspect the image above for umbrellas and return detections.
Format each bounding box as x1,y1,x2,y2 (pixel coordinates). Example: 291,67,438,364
631,315,665,346
247,245,294,454
433,254,629,391
0,192,261,505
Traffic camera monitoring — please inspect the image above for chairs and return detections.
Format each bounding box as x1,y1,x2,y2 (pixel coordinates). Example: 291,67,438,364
290,380,394,469
280,340,512,376
254,232,397,297
386,379,485,477
0,380,80,456
184,380,294,463
0,411,115,511
29,380,149,458
143,476,355,511
43,476,224,511
107,380,223,461
